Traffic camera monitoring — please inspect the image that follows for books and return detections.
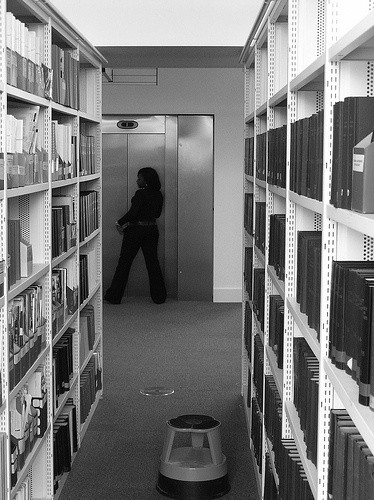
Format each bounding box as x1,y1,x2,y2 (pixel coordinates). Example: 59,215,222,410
0,10,107,498
237,90,374,500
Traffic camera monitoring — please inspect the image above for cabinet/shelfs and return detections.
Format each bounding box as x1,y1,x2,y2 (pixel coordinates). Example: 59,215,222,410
242,0,373,499
7,0,109,500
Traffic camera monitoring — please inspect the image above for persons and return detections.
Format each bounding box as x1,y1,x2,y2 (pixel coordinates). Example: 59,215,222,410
101,164,169,304
